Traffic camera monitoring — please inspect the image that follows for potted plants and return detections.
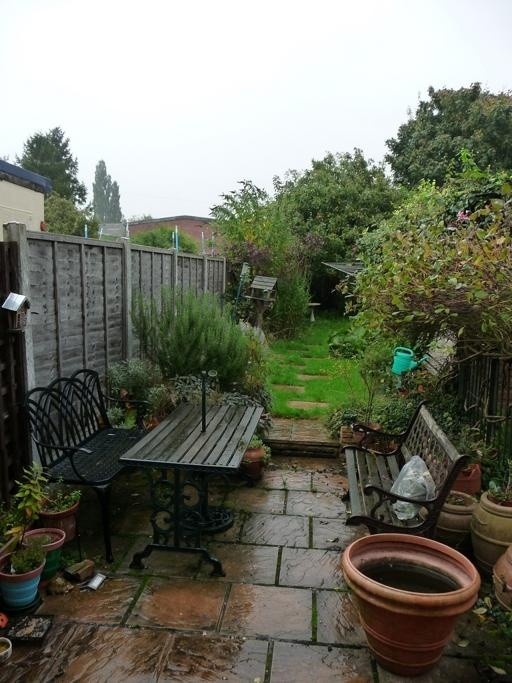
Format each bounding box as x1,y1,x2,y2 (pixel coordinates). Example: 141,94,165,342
1,457,81,607
243,433,271,484
428,391,511,577
352,344,393,449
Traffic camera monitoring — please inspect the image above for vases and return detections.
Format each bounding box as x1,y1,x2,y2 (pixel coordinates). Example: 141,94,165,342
340,534,483,677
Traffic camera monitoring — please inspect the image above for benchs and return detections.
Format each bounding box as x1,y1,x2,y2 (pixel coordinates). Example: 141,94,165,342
341,398,472,540
22,368,166,564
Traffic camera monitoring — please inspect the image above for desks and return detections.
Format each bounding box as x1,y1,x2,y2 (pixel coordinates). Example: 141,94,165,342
118,404,266,580
